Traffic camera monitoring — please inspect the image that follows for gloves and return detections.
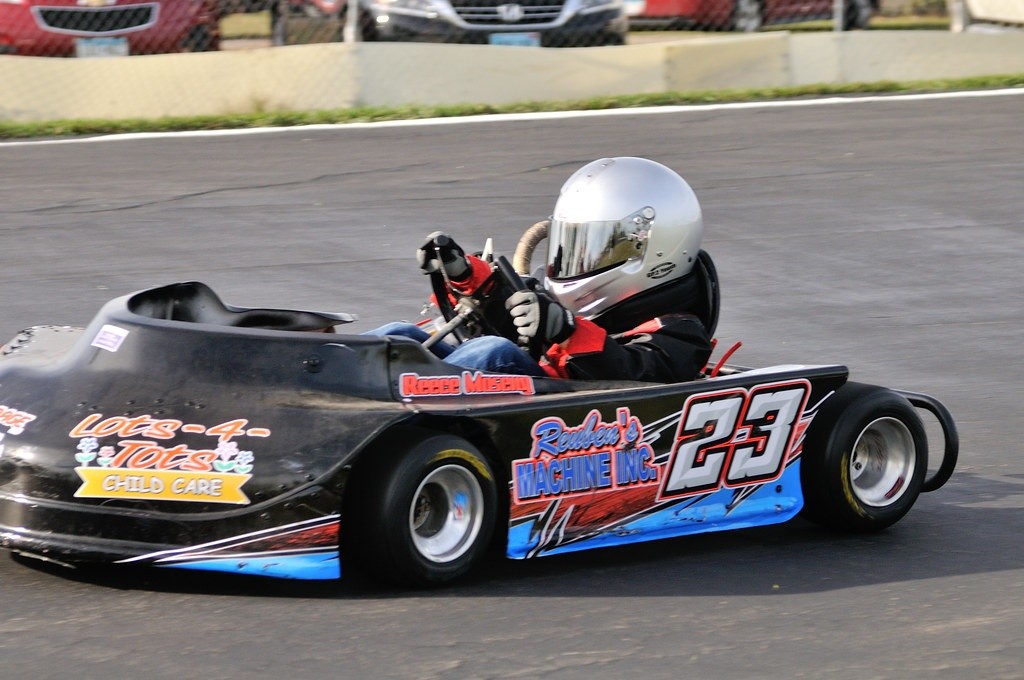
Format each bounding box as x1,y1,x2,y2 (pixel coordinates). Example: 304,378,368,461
504,289,574,346
417,229,473,282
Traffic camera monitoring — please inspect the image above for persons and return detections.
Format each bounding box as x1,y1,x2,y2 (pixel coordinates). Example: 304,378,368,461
416,156,715,386
608,232,616,259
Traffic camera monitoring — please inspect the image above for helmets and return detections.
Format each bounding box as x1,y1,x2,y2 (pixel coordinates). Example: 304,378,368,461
543,156,704,323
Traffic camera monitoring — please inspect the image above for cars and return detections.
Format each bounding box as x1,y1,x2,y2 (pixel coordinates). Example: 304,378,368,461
0,0,874,56
